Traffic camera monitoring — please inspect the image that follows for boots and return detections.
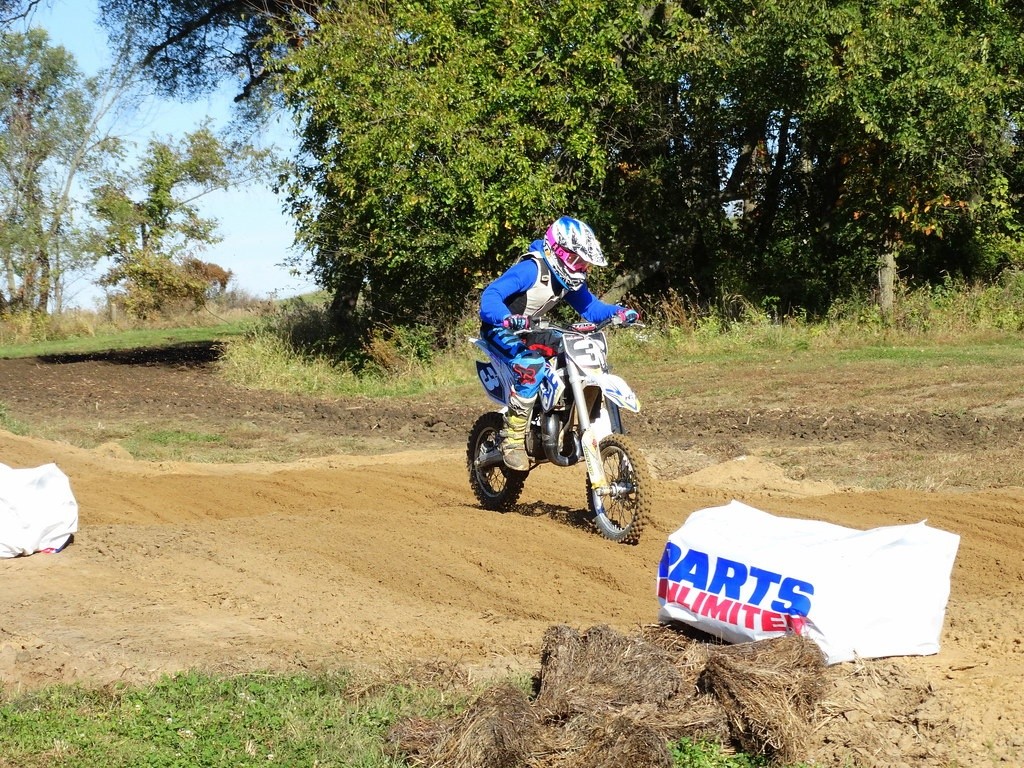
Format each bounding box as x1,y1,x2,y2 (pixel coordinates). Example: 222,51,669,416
498,392,539,472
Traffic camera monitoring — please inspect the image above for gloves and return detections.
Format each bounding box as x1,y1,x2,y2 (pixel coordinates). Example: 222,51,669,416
503,314,529,330
616,308,640,323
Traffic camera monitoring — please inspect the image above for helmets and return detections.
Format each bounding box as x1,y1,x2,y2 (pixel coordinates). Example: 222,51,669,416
541,215,608,293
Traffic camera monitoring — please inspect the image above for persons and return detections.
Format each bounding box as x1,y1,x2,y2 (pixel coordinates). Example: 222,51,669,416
477,216,641,472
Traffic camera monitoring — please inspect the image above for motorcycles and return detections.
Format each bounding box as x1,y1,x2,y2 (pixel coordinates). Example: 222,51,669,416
464,312,652,547
